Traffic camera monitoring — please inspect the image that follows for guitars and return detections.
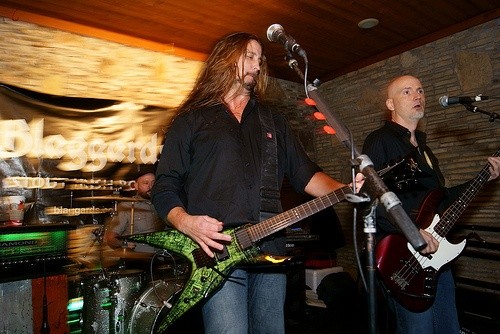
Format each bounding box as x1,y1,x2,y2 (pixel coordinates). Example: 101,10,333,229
372,150,500,314
114,158,404,334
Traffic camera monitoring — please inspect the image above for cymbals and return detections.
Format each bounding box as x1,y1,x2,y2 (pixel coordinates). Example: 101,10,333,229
75,196,150,202
99,207,153,213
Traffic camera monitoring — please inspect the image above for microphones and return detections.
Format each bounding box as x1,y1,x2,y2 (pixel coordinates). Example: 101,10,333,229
439,95,489,107
266,23,306,56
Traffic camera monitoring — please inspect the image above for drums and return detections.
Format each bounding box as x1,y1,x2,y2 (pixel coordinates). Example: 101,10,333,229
128,277,209,333
80,269,145,333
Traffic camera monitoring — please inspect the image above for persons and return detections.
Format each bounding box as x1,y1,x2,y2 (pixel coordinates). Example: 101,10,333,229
103,163,168,253
361,76,500,334
150,32,365,334
